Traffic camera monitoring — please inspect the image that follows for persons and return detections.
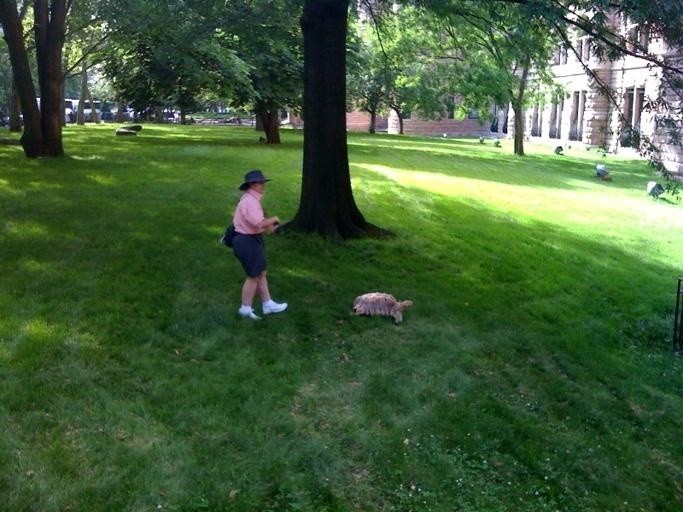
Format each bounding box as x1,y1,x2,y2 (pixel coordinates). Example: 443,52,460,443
233,170,288,320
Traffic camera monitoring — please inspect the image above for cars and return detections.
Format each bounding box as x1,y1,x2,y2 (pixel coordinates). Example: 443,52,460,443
0,94,177,129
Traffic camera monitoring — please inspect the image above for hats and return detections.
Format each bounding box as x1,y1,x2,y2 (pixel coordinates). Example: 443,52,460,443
239,171,273,191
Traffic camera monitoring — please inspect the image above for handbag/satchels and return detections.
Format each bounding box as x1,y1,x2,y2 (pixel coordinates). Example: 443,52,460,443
223,224,237,248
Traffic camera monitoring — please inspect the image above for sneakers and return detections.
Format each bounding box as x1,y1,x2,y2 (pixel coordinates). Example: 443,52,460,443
261,301,287,314
238,307,263,320
